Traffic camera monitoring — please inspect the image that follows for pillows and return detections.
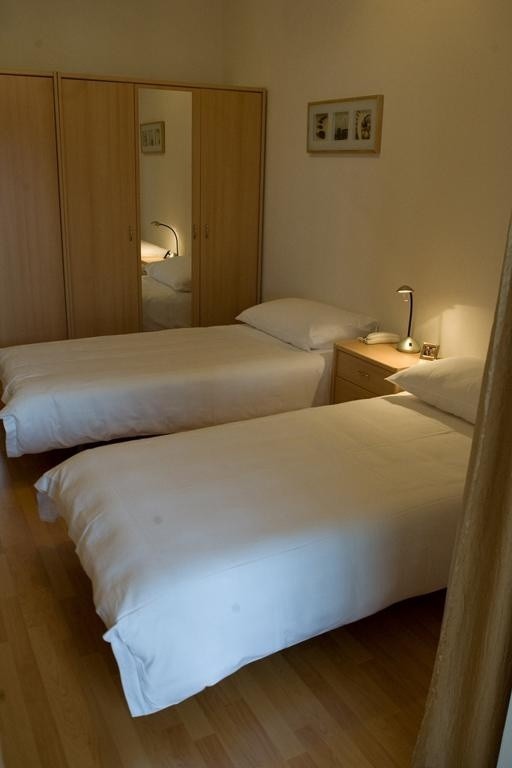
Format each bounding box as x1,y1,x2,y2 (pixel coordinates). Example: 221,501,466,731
146,256,191,292
384,354,486,426
235,296,379,353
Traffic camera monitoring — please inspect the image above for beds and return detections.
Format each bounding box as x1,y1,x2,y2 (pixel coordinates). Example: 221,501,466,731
0,295,381,461
139,256,194,331
35,351,489,718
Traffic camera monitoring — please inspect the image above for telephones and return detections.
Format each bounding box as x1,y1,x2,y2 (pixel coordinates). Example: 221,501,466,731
364,331,401,345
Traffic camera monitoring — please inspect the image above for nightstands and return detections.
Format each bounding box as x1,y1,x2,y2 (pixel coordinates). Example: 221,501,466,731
139,254,180,277
331,335,440,405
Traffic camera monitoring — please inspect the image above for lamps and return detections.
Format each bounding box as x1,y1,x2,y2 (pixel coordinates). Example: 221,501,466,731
150,219,179,257
396,284,423,353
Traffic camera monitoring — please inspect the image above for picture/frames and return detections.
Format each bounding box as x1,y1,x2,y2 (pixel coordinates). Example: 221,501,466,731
420,342,440,361
163,249,171,259
306,94,385,154
139,121,166,154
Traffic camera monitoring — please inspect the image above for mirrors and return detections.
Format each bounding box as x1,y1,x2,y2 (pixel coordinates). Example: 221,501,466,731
136,84,195,330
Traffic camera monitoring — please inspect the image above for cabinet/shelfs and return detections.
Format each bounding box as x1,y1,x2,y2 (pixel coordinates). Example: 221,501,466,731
1,68,68,350
58,73,270,342
